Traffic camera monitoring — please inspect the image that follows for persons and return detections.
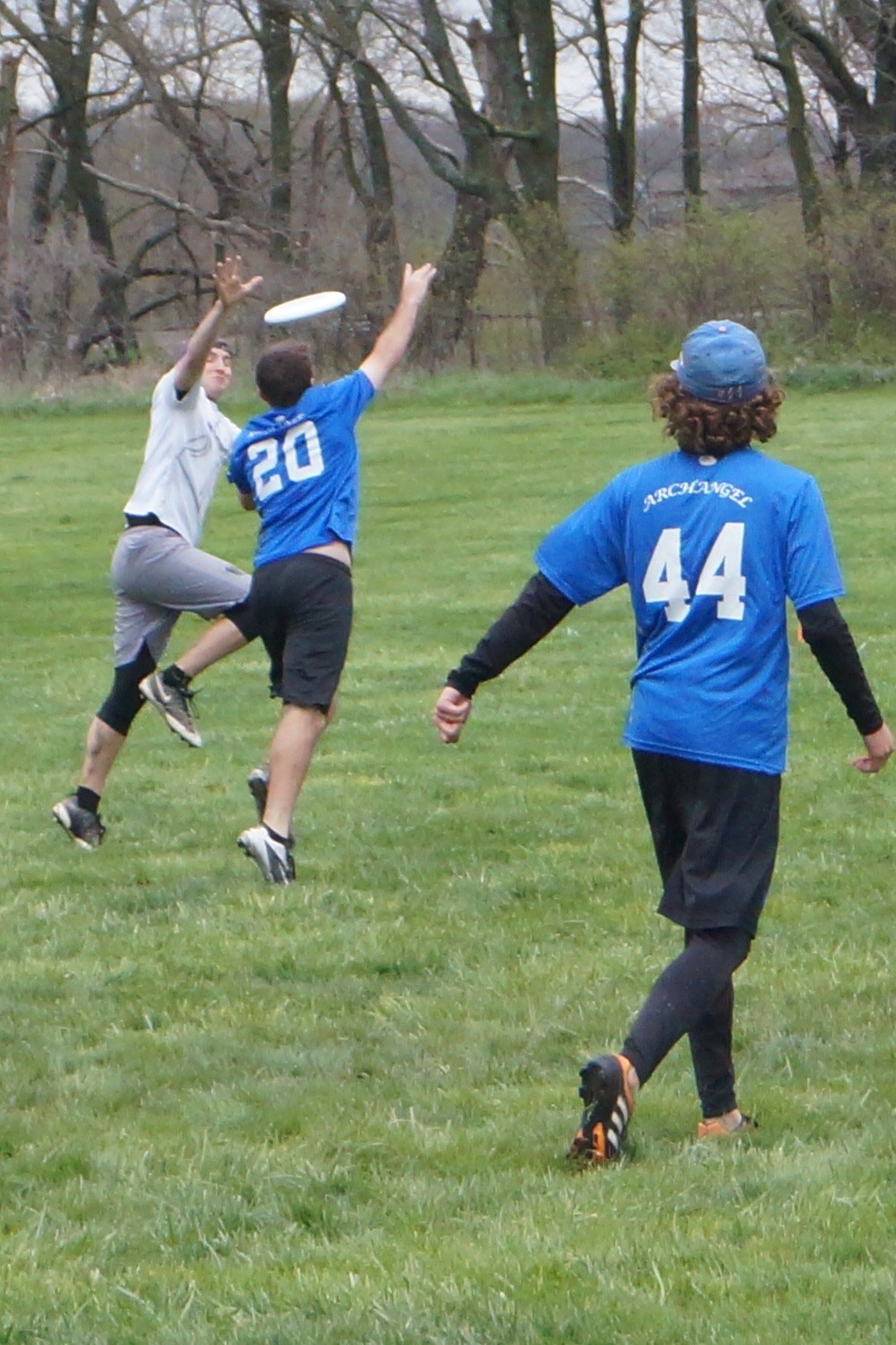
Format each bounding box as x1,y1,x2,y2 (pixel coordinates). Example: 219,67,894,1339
12,267,74,339
225,263,437,887
54,255,264,851
432,318,896,1173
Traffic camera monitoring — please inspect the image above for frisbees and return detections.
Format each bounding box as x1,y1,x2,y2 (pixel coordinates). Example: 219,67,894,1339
264,290,347,324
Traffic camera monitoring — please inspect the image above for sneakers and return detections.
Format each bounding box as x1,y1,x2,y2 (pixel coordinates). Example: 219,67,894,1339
237,826,297,888
565,1054,635,1173
699,1115,761,1140
246,764,267,823
139,671,203,748
53,795,106,852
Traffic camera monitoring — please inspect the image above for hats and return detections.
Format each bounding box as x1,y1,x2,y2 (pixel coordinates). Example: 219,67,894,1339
670,320,767,402
212,340,233,354
